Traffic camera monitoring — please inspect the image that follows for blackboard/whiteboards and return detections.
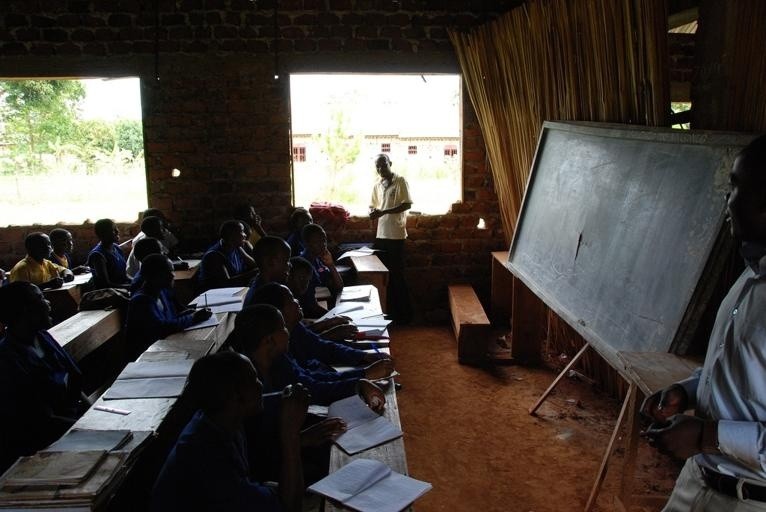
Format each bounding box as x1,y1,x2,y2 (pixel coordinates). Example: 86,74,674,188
505,119,759,381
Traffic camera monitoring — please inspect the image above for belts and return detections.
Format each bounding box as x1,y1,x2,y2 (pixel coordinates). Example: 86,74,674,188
697,466,761,503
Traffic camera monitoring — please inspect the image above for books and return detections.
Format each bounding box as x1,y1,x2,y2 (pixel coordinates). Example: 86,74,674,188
307,456,432,512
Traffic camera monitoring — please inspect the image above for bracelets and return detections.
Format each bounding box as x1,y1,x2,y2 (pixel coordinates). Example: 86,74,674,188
694,417,712,457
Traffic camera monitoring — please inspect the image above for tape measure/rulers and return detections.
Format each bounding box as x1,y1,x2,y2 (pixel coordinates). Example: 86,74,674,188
93,405,131,415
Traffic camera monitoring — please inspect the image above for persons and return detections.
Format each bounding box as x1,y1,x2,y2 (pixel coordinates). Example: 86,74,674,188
368,154,414,326
638,134,766,512
2,202,404,510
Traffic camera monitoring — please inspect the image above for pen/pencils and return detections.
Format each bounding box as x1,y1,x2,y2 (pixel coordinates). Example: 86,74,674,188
56,270,60,279
333,313,356,324
177,256,184,262
204,293,208,307
371,343,382,359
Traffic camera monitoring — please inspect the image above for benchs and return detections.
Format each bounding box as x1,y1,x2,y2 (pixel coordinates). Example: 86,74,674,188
447,280,494,366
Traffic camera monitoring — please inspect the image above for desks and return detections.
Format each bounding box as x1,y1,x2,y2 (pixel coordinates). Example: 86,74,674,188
491,250,545,364
613,348,710,512
